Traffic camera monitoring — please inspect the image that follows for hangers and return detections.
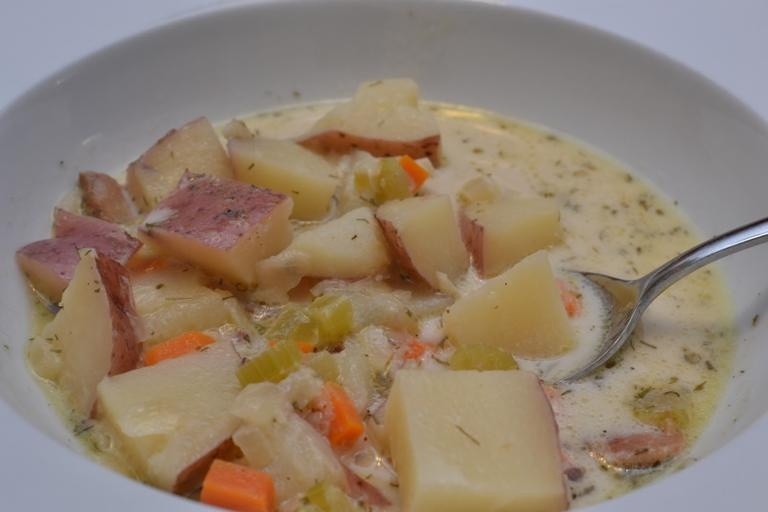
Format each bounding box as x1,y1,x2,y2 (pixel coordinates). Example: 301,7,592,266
475,215,768,384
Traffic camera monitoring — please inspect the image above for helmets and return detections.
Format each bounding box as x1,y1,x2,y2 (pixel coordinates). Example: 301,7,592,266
1,0,768,512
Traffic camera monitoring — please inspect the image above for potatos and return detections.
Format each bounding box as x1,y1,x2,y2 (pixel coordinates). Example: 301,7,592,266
14,75,576,510
